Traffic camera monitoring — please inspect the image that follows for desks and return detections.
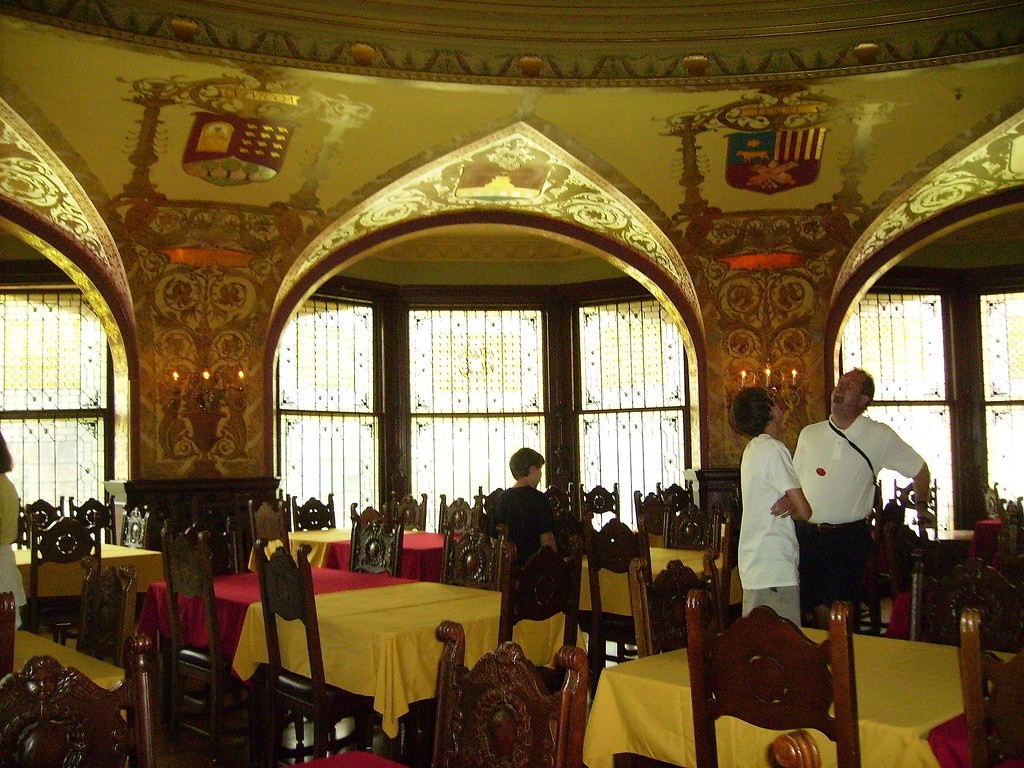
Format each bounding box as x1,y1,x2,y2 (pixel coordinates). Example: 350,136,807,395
582,627,1016,768
320,532,479,585
13,630,130,768
137,567,418,768
232,582,587,768
11,544,164,673
867,525,973,646
577,546,744,705
247,528,419,572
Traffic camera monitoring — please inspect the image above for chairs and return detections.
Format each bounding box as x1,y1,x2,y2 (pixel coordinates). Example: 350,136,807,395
0,480,1024,768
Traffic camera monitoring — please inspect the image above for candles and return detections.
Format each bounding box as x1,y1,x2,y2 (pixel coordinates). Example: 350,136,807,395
238,371,244,389
203,370,209,389
765,369,770,387
741,370,746,387
791,369,798,388
173,371,179,389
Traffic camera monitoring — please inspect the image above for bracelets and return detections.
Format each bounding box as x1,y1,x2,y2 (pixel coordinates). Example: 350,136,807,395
916,501,928,509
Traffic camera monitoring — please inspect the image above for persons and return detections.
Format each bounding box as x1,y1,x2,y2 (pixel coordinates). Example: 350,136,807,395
728,386,812,627
0,431,27,631
792,366,937,635
491,448,571,579
